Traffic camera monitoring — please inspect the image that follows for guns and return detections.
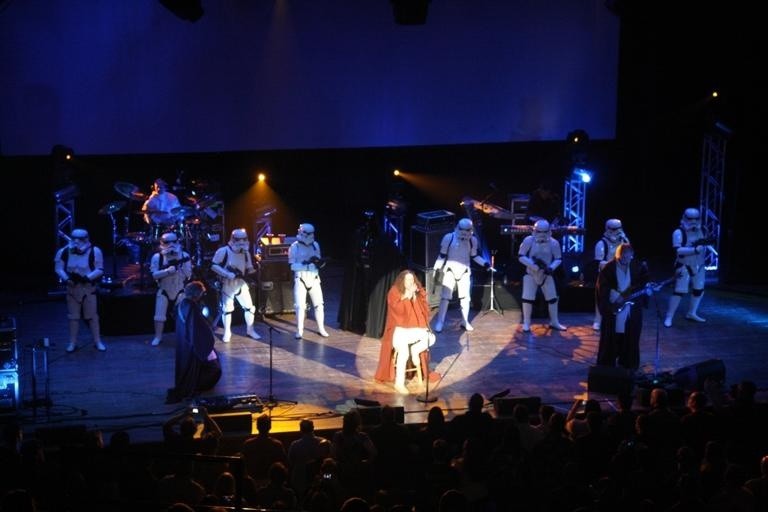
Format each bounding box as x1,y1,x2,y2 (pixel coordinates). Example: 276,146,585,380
58,271,96,287
160,256,190,270
689,233,717,248
301,256,332,272
532,256,550,275
226,265,249,283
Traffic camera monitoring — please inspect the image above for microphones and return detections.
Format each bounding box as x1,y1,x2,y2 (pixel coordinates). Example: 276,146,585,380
214,281,222,292
642,261,651,274
415,289,420,294
173,186,186,190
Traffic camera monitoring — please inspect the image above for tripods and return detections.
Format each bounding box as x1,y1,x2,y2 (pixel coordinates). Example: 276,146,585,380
242,334,298,408
479,256,505,318
103,218,225,289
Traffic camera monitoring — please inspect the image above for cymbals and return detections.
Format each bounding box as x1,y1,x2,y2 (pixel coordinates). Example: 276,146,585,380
170,205,194,216
114,182,149,201
137,209,169,214
98,200,127,214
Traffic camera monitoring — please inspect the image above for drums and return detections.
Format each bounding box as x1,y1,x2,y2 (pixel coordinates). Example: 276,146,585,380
190,229,221,260
124,232,148,264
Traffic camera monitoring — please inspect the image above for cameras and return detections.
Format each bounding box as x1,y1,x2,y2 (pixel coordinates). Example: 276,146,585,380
190,408,201,414
322,472,332,480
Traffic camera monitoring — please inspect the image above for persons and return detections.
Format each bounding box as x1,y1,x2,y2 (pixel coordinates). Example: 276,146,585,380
149,233,193,345
286,224,329,339
518,221,568,332
594,218,630,330
210,229,262,342
2,388,767,512
662,208,707,326
429,218,498,331
595,243,652,378
373,268,441,396
142,177,186,227
165,280,224,405
55,228,108,351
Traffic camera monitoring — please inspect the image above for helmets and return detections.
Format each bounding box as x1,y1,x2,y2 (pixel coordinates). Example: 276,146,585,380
159,233,179,254
680,208,700,230
603,219,625,241
454,219,473,240
228,228,248,251
68,229,90,254
296,223,314,246
532,220,551,242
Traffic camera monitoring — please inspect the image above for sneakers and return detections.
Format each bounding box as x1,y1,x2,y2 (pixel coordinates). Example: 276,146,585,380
393,384,409,395
410,345,420,366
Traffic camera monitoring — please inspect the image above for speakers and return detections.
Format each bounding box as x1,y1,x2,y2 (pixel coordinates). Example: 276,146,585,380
589,366,635,393
351,406,404,425
672,359,726,390
494,397,540,415
204,412,252,434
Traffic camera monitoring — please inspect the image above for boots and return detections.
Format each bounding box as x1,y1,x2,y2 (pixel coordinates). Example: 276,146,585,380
244,311,260,339
433,300,449,332
522,303,531,331
88,320,105,350
592,304,601,330
663,295,681,327
65,320,78,352
548,302,566,331
151,321,163,346
295,310,304,338
685,294,705,322
460,299,473,331
314,309,328,337
222,314,231,343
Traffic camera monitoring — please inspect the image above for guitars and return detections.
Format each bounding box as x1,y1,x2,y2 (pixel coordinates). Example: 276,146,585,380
607,272,684,315
